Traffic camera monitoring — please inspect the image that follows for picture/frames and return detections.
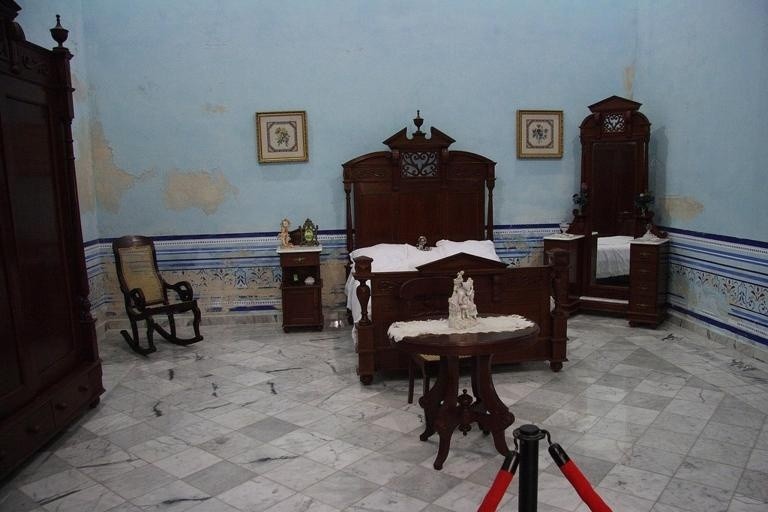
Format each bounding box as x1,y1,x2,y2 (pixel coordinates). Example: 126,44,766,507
516,110,564,158
256,111,308,164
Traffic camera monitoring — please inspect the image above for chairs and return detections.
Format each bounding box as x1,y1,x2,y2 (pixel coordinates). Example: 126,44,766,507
400,278,478,404
111,236,203,355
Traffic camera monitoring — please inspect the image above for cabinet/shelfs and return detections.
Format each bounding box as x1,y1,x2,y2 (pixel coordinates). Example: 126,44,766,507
544,95,670,329
2,0,104,484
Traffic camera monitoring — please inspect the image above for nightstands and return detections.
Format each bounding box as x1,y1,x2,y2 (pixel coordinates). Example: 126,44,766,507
276,245,325,332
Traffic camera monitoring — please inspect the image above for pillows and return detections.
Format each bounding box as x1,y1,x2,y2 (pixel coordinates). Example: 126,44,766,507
349,238,501,273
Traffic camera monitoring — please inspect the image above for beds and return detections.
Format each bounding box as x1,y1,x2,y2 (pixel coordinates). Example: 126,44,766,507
342,108,568,386
